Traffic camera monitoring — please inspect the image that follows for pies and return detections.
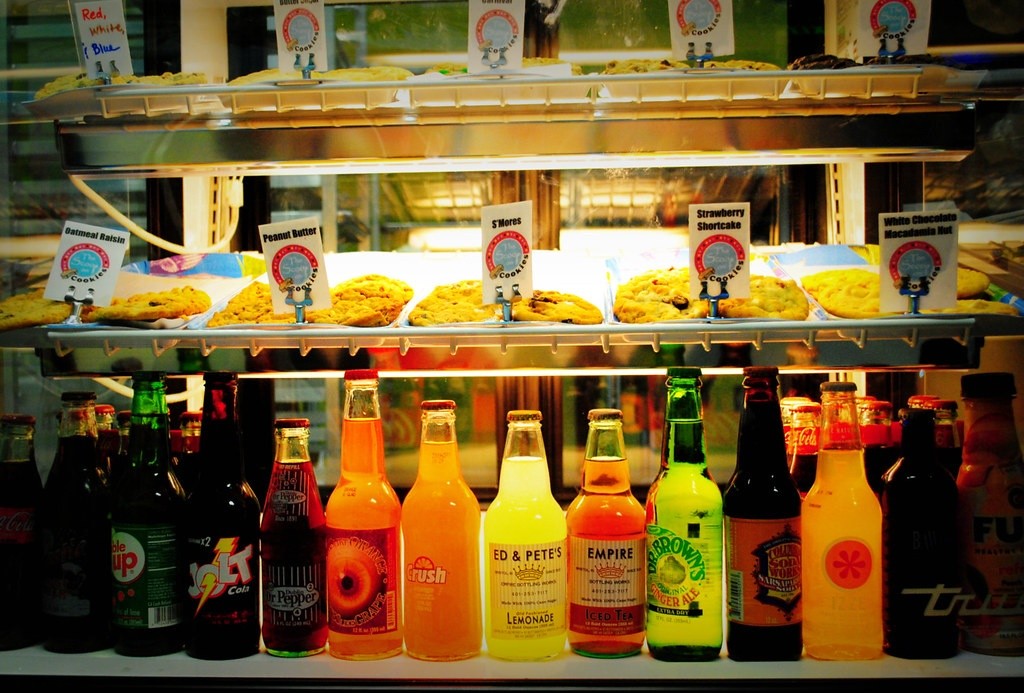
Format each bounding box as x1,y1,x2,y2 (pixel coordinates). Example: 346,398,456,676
34,51,951,100
0,263,1015,326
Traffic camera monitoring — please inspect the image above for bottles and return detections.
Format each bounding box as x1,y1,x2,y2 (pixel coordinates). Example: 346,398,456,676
402,400,482,661
0,412,44,651
261,419,328,656
326,370,403,660
565,409,646,657
484,410,567,659
723,364,1024,662
44,370,260,660
643,366,723,661
